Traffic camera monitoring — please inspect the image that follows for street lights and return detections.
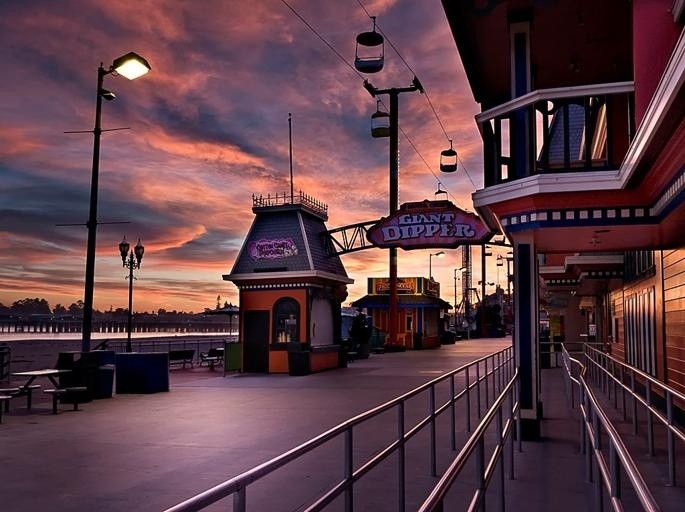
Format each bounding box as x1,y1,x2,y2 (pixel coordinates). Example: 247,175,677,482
453,267,468,329
55,52,152,354
120,233,143,351
480,281,495,301
428,251,444,279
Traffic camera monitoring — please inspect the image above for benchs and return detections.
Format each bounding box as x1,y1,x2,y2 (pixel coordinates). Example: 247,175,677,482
169,350,194,369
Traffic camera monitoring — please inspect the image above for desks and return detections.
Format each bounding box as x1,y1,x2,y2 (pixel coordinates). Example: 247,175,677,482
6,368,73,414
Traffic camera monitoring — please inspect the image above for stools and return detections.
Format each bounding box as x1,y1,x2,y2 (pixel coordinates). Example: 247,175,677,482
0,396,12,424
19,385,42,411
0,387,20,412
201,347,224,371
43,389,66,415
66,386,90,411
